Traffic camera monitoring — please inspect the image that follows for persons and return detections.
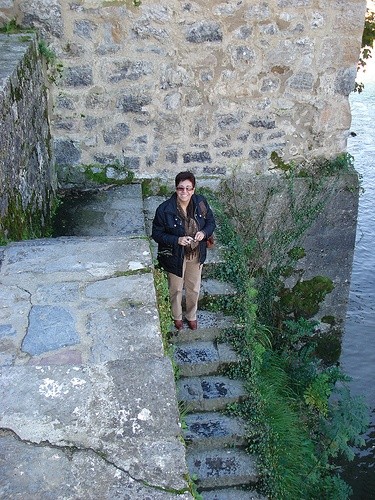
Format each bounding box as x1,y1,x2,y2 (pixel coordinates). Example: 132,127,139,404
151,172,215,331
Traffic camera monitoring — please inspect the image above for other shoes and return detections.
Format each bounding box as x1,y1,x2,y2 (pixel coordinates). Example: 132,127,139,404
175,320,183,329
188,320,198,330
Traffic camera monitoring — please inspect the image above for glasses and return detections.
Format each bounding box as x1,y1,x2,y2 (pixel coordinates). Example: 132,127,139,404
175,186,193,191
